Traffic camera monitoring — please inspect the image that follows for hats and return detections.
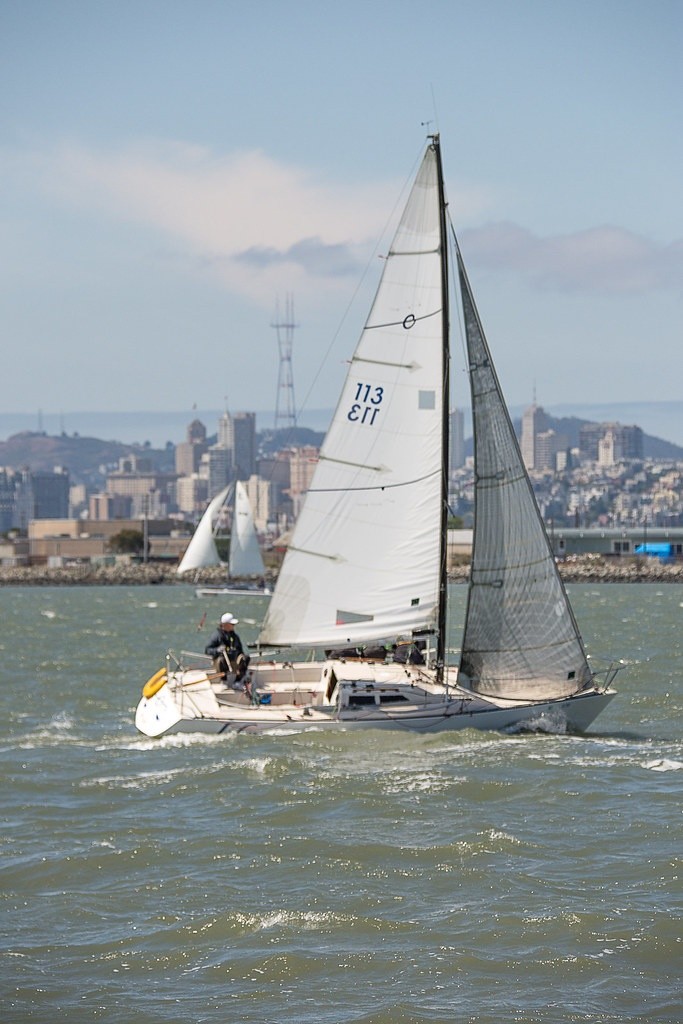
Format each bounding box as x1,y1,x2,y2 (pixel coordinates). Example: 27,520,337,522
221,612,239,626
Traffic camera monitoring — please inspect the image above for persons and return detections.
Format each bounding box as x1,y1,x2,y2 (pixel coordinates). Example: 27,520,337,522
205,612,250,689
325,639,427,665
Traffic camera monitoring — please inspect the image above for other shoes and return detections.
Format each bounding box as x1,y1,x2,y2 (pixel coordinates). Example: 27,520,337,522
231,681,245,691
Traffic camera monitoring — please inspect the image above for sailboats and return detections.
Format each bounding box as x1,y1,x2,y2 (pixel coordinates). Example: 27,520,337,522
134,113,618,742
177,481,275,597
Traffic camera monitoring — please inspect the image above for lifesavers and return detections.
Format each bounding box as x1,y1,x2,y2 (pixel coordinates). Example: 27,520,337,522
143,666,170,699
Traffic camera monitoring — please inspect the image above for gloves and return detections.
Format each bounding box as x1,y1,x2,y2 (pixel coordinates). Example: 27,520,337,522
217,645,226,653
